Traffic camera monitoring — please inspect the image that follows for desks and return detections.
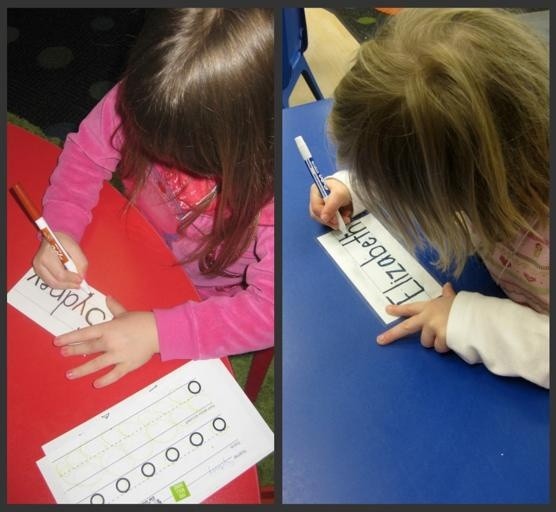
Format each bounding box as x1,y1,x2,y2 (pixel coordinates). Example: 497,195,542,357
283,96,549,505
7,120,261,504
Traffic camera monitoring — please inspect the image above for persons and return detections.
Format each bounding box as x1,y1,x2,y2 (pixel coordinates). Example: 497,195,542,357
32,8,277,389
309,3,549,394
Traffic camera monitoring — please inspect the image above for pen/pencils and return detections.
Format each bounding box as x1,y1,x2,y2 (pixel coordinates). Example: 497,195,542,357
11,181,94,297
294,135,348,239
